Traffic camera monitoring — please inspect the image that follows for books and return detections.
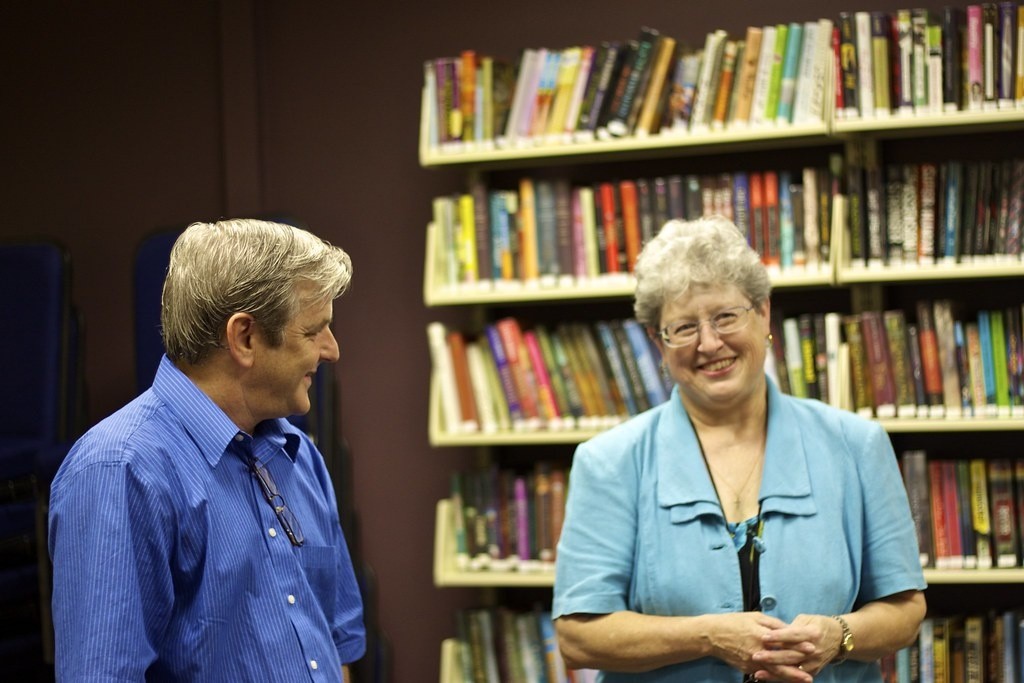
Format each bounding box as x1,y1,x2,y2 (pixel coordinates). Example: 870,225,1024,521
423,0,1024,683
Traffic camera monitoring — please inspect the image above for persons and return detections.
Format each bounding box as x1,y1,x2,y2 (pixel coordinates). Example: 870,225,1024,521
48,218,366,683
551,213,928,683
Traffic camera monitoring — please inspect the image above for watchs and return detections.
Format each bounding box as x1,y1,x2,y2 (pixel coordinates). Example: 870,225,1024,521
831,614,855,667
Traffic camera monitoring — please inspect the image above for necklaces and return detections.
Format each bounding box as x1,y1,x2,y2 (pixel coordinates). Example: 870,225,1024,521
695,436,764,511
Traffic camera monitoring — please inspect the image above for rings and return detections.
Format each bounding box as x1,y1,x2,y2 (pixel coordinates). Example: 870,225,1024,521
799,665,802,669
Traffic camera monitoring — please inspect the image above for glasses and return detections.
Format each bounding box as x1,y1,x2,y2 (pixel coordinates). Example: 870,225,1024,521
249,456,304,547
656,303,754,348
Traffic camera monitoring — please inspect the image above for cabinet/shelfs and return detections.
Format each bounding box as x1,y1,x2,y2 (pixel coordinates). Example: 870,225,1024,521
418,91,1024,683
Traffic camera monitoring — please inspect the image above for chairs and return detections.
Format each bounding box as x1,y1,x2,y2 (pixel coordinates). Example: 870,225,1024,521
131,222,395,683
0,236,88,683
